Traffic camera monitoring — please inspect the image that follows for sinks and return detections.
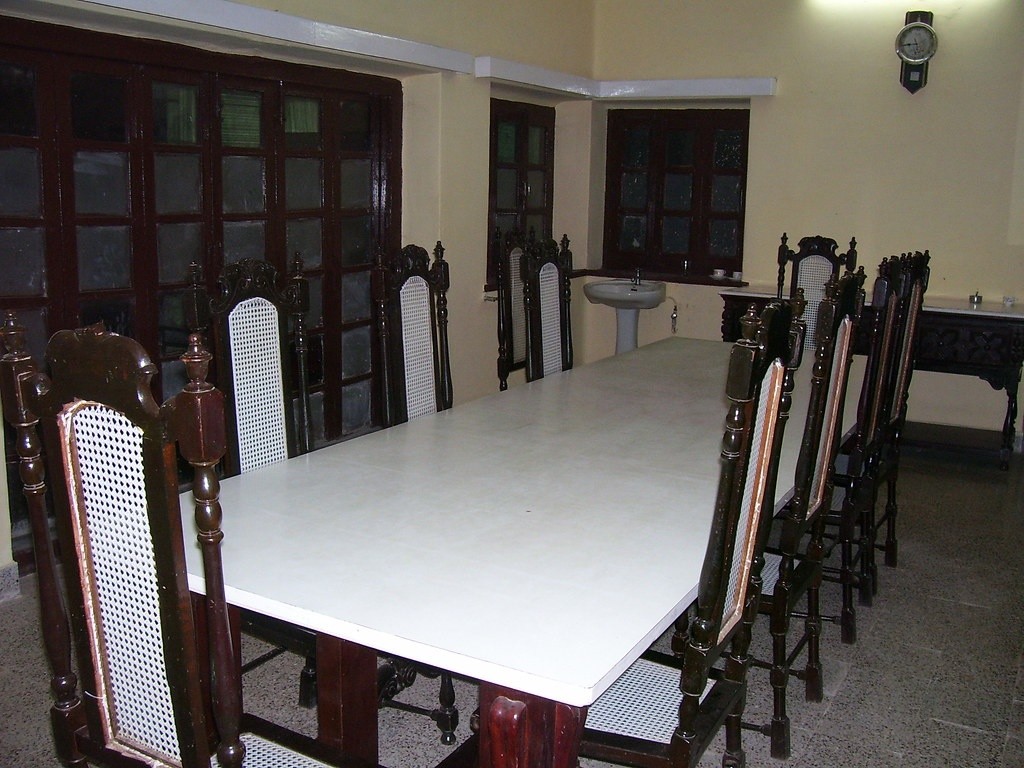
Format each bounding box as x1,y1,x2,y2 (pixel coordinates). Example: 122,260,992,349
583,278,667,310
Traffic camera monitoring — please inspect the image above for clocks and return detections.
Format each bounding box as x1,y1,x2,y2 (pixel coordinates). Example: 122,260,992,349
895,22,938,66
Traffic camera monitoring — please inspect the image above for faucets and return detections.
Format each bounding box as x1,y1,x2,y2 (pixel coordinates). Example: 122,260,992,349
631,267,640,285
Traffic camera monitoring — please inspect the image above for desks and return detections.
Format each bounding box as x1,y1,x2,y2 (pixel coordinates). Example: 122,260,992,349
719,287,1024,472
180,335,866,767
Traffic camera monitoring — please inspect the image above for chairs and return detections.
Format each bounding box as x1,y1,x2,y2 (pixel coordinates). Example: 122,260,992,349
0,225,929,768
